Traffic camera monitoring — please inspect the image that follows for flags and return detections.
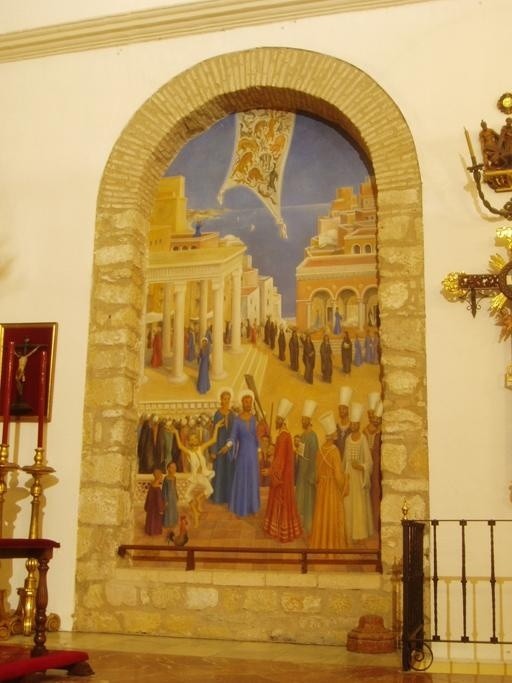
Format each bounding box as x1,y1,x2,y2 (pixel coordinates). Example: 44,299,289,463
216,104,295,238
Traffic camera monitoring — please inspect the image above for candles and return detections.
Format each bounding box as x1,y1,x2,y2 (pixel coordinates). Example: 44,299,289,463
2,341,14,445
463,126,477,166
38,351,47,448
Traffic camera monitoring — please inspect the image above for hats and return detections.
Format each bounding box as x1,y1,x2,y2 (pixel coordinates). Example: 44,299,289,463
318,410,337,435
368,393,379,410
338,386,351,408
349,403,364,422
277,398,294,420
302,400,317,417
374,401,383,418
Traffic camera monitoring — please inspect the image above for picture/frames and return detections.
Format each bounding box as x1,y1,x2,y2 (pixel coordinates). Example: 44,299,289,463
0,322,59,423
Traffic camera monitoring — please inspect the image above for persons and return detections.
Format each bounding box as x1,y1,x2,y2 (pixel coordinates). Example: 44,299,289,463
497,115,511,170
192,218,205,236
11,346,40,395
477,117,500,171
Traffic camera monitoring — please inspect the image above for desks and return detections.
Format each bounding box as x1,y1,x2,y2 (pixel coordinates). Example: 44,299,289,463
0,539,60,655
